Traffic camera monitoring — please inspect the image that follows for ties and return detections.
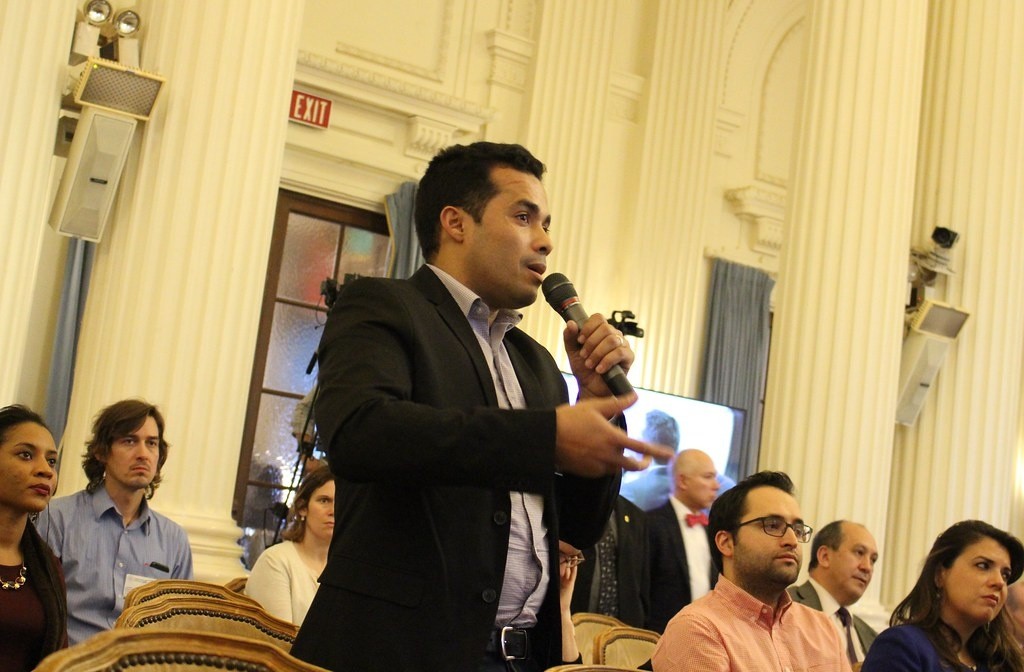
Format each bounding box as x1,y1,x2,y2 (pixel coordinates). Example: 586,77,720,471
837,607,857,665
598,520,620,618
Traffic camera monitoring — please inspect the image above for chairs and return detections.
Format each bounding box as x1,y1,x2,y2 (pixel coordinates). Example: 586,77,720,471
31,576,665,671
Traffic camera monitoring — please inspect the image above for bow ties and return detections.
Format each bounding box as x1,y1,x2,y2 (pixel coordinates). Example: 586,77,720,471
685,513,708,528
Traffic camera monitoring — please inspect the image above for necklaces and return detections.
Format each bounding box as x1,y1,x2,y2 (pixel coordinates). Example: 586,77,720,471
0,550,27,590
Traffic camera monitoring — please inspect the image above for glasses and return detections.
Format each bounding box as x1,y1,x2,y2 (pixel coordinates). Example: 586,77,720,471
726,517,813,543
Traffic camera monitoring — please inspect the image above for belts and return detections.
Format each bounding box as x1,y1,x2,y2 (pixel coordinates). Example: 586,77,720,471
483,626,537,660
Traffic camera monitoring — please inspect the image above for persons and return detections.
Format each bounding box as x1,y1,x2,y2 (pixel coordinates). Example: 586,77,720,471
1,404,69,672
242,382,336,626
619,410,737,512
559,448,721,665
787,520,879,672
650,470,853,671
38,399,194,647
288,141,675,671
861,520,1024,671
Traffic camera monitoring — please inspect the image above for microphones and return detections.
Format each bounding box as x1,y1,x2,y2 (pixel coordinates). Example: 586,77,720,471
542,272,633,397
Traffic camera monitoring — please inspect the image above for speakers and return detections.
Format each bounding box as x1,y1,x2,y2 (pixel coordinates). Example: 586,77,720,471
894,328,950,427
47,105,137,243
